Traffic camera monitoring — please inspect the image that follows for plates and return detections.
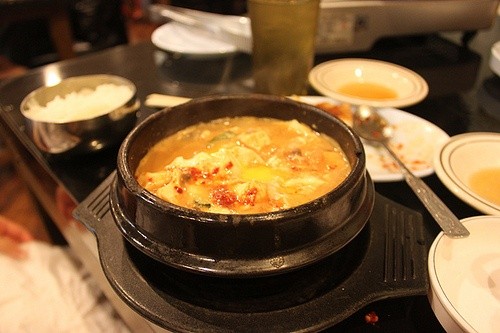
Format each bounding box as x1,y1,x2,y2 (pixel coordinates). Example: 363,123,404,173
151,14,254,56
435,132,500,215
490,42,500,75
428,216,500,333
309,58,429,107
289,96,449,182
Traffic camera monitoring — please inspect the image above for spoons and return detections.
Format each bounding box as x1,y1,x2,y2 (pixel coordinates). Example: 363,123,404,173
353,105,470,238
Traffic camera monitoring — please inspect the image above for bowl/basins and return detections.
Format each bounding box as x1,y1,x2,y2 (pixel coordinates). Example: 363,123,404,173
110,97,375,273
20,75,140,155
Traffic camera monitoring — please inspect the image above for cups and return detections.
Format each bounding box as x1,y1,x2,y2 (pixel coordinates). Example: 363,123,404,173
248,0,319,96
